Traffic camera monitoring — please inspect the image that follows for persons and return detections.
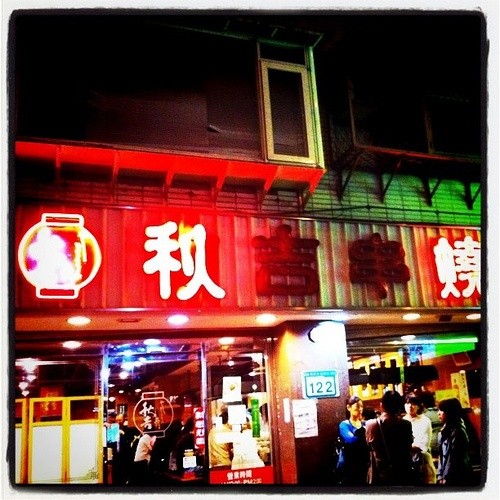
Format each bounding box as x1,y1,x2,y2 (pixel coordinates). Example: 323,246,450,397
400,392,439,485
457,407,485,489
436,397,474,489
365,390,416,486
334,395,374,485
101,403,209,490
417,389,446,462
